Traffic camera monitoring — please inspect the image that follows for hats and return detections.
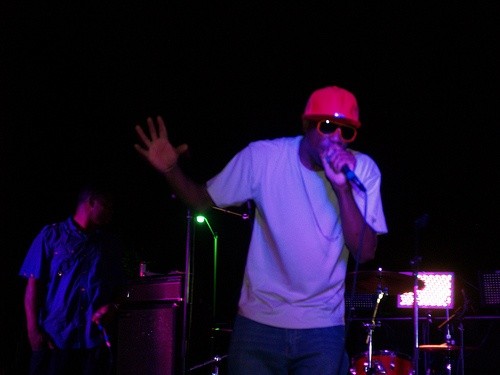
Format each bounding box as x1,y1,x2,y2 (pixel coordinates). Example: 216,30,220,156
304,86,360,128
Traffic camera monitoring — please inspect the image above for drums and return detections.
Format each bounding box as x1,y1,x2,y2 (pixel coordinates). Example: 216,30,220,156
352,349,413,375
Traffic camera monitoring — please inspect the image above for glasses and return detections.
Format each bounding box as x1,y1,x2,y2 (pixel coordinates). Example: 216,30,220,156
317,119,357,143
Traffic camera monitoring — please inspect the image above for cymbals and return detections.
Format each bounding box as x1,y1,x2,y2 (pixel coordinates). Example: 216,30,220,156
418,344,462,352
345,269,425,297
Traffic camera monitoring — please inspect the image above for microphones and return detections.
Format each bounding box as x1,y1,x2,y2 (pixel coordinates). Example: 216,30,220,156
327,156,367,192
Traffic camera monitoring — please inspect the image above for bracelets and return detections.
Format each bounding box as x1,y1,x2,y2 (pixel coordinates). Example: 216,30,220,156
109,300,120,314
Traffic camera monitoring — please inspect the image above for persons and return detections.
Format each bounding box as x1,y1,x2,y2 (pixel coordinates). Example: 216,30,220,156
135,86,388,375
18,186,138,375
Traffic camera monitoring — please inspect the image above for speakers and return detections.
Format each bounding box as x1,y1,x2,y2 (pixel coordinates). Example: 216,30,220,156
113,305,183,375
